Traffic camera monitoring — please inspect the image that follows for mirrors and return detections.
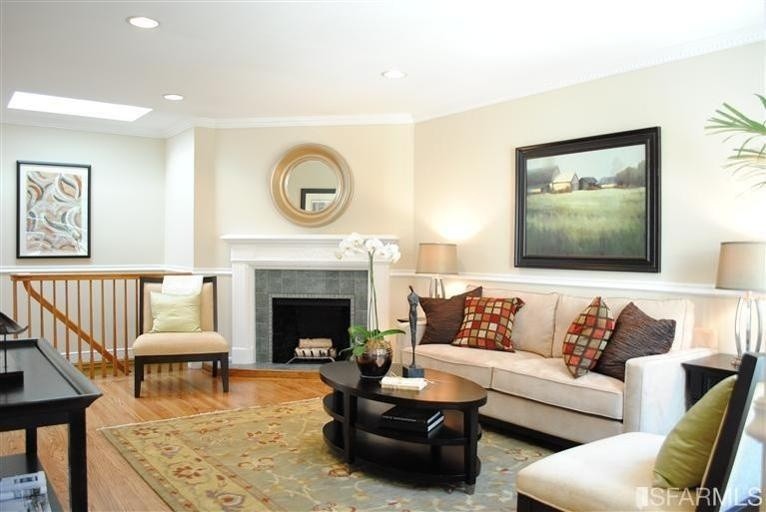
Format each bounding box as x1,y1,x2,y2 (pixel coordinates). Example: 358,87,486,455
266,140,357,230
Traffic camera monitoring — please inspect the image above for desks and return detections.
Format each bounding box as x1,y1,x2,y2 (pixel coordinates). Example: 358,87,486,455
680,350,747,415
0,332,105,512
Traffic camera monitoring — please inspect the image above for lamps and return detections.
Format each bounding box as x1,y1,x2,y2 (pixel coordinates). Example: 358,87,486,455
713,239,766,369
412,240,464,302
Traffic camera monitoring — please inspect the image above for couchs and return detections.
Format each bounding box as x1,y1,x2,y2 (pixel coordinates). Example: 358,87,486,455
398,279,714,449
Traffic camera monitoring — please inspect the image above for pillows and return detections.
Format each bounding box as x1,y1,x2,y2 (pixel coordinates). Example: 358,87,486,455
591,299,678,383
147,289,206,336
450,294,526,354
646,369,739,493
408,282,485,347
559,295,620,380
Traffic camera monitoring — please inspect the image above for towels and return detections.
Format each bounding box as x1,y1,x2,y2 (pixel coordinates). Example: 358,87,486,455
160,274,205,297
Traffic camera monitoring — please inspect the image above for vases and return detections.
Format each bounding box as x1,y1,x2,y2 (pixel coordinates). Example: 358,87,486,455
355,338,396,380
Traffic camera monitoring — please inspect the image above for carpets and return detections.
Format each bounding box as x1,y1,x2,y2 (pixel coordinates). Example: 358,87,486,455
93,391,576,511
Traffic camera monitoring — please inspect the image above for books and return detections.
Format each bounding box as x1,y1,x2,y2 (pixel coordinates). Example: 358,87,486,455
381,407,444,433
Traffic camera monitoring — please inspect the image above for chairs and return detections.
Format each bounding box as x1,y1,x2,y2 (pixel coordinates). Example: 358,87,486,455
514,347,766,511
130,274,232,399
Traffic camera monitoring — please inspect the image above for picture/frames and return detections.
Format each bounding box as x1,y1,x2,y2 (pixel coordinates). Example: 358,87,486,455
511,123,667,275
14,160,94,261
298,187,336,215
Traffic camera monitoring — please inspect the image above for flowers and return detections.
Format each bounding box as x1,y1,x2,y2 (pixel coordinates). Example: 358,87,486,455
334,230,407,358
